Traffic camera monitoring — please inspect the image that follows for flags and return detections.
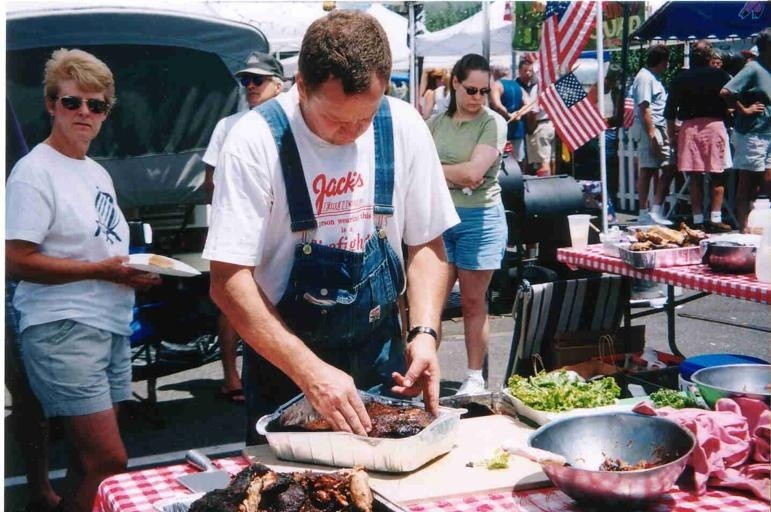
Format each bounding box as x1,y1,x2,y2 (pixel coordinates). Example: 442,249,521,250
534,2,595,91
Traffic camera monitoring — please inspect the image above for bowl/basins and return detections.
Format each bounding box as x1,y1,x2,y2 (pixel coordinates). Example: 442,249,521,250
697,237,758,275
528,409,699,511
690,363,771,410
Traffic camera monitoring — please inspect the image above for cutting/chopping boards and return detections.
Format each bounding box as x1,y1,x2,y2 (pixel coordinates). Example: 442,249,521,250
359,413,552,505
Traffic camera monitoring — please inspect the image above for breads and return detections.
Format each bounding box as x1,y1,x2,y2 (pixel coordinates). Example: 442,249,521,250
148,255,176,271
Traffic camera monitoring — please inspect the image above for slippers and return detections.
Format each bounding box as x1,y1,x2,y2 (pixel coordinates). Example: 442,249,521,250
218,387,246,405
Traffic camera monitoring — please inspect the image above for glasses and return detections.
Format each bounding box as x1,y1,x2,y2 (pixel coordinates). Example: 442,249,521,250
464,85,490,95
60,95,107,113
241,75,263,85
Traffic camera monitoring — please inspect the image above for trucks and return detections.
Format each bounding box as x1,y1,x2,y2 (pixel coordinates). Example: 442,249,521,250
6,9,278,369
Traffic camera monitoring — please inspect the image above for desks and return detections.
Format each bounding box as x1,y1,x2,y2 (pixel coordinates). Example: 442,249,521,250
91,397,771,512
557,242,771,365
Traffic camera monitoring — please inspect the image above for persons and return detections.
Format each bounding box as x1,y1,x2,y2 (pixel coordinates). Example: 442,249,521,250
3,281,66,512
199,49,292,404
421,52,508,399
220,6,463,447
417,24,771,236
5,44,169,512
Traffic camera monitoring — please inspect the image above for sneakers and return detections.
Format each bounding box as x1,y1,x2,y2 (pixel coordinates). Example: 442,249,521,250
709,221,732,232
637,211,673,227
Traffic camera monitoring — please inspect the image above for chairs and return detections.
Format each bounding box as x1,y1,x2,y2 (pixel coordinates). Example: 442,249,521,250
521,176,598,282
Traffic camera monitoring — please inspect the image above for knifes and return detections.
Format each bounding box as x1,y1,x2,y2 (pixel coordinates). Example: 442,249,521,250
177,447,237,498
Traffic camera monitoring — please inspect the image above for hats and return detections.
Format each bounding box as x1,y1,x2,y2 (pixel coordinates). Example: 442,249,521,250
234,51,283,80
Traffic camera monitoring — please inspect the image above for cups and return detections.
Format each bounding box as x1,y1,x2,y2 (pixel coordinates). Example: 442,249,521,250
567,213,590,252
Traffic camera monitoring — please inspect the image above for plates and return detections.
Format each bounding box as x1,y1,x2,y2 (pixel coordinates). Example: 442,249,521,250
120,251,203,283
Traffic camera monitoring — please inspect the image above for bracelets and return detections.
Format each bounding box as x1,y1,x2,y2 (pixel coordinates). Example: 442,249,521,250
405,325,437,347
